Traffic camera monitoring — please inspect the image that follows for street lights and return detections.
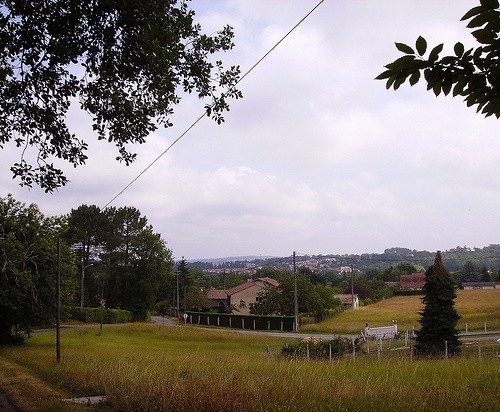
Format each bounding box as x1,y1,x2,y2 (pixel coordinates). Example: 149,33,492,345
79,261,94,313
